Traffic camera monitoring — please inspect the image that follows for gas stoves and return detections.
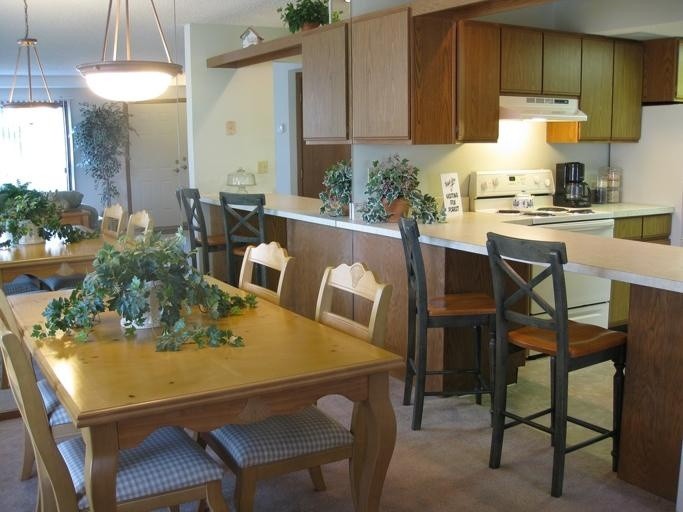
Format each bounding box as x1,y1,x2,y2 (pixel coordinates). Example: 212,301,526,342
469,169,614,225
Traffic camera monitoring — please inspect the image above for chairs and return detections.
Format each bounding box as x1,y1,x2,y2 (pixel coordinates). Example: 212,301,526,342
177,188,235,275
195,261,394,512
218,192,269,290
100,202,125,239
485,232,627,498
238,241,296,306
126,209,154,244
398,216,497,430
0,289,60,512
0,318,229,512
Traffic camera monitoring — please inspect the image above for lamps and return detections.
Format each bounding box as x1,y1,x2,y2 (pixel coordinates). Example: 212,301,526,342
1,1,184,127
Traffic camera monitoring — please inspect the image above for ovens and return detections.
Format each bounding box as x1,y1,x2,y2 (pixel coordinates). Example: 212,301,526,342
530,219,616,315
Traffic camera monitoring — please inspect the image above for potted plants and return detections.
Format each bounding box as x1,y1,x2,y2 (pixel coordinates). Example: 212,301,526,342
0,181,98,247
355,154,448,225
318,159,354,217
277,1,343,32
30,227,259,351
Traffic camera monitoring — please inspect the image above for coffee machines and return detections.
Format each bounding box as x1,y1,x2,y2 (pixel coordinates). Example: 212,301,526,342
553,162,592,208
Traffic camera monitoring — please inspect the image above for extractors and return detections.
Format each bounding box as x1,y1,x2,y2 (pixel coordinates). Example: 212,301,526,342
499,96,589,122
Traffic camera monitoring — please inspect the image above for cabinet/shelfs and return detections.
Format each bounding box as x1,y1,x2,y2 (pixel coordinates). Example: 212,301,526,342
610,213,672,329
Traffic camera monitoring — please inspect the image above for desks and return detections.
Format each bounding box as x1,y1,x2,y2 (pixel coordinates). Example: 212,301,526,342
0,233,123,390
59,210,91,226
9,274,406,512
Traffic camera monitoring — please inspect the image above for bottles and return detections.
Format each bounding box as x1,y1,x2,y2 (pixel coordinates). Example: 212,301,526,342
597,168,621,204
